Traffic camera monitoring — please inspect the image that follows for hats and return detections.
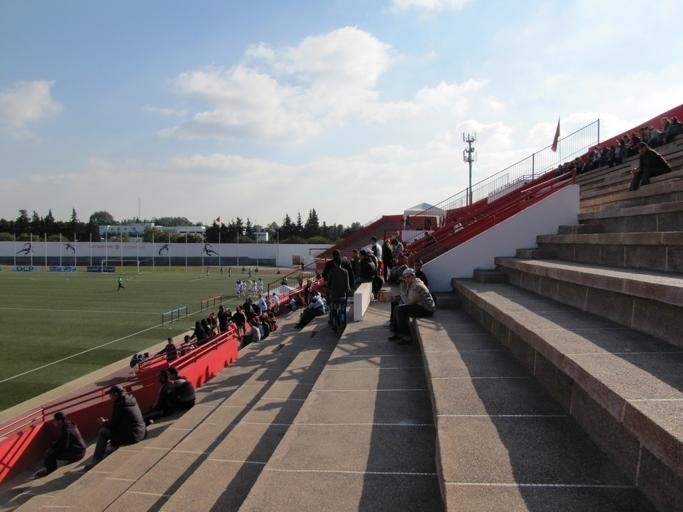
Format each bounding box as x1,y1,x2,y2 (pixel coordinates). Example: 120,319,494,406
105,384,124,395
402,268,416,278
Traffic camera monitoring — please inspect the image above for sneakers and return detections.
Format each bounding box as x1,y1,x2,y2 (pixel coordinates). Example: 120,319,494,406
383,320,413,345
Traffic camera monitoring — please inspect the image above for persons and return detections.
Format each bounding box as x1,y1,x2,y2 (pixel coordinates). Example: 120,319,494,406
554,116,683,192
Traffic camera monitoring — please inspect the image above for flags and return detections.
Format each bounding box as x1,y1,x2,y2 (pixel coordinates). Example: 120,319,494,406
551,118,561,153
